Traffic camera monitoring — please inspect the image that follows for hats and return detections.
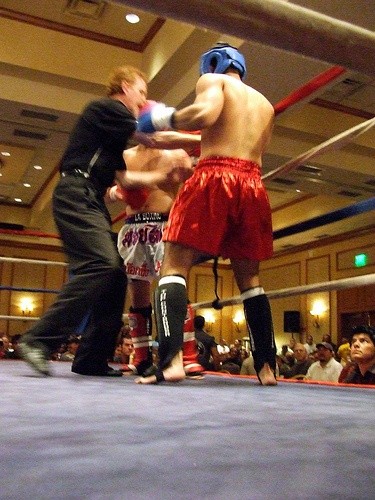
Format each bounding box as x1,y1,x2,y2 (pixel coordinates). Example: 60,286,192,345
316,342,333,351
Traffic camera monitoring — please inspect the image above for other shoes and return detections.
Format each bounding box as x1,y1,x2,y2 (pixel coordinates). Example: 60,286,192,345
70,366,123,377
17,343,49,376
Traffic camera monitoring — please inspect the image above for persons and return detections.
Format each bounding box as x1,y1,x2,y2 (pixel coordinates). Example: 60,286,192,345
0,314,375,388
104,99,205,377
14,64,202,377
136,42,278,388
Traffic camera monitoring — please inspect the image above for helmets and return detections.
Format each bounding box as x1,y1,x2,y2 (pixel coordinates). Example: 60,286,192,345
200,42,246,80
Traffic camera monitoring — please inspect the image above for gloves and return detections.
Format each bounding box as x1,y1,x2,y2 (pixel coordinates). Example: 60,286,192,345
137,100,176,133
